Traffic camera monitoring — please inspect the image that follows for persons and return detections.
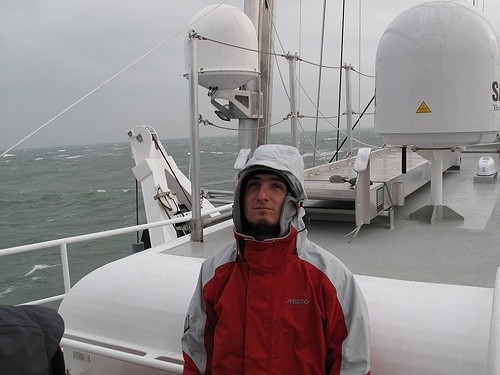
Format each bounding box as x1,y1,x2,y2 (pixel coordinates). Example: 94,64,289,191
180,143,371,375
0,305,68,375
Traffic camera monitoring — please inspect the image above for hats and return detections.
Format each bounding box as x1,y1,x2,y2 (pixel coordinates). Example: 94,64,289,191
237,144,308,201
